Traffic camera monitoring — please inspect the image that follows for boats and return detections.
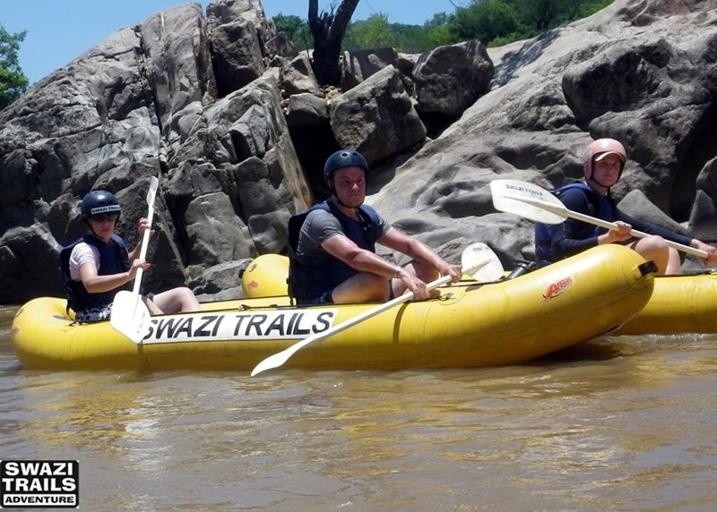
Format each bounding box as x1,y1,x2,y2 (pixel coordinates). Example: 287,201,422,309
430,268,717,336
13,233,654,375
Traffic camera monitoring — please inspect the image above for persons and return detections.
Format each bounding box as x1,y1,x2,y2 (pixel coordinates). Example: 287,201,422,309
285,147,465,308
533,136,717,277
56,188,200,328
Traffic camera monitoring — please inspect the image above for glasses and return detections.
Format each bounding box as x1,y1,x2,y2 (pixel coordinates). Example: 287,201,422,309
91,214,118,222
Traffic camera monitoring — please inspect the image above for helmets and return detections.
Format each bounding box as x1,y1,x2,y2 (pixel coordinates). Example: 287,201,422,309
81,190,122,223
583,138,627,180
324,150,369,180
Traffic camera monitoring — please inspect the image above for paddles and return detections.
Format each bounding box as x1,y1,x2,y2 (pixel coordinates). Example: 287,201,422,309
491,178,709,259
249,242,506,378
110,176,159,343
